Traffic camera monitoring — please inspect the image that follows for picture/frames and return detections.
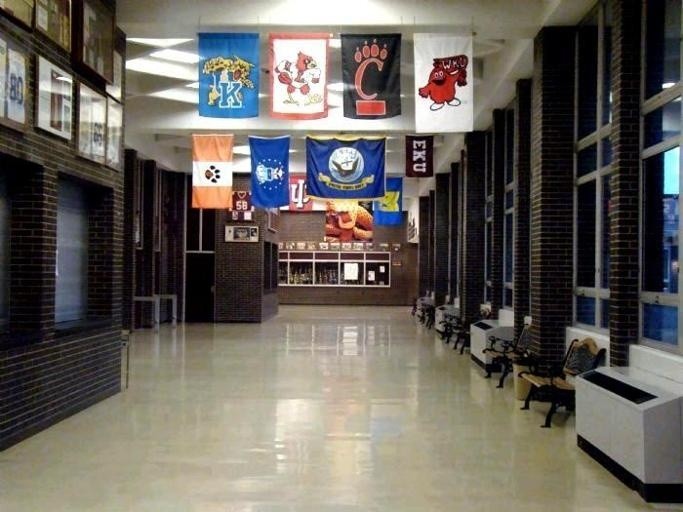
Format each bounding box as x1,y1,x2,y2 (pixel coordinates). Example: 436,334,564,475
1,0,130,173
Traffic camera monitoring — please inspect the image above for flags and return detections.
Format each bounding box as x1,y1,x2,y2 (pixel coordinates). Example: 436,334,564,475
268,31,331,119
372,177,404,227
190,133,234,209
247,134,291,208
305,134,386,200
413,32,474,134
196,31,260,118
399,133,435,198
341,32,401,119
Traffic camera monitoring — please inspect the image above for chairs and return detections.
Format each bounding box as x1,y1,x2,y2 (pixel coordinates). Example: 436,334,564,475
409,281,606,430
